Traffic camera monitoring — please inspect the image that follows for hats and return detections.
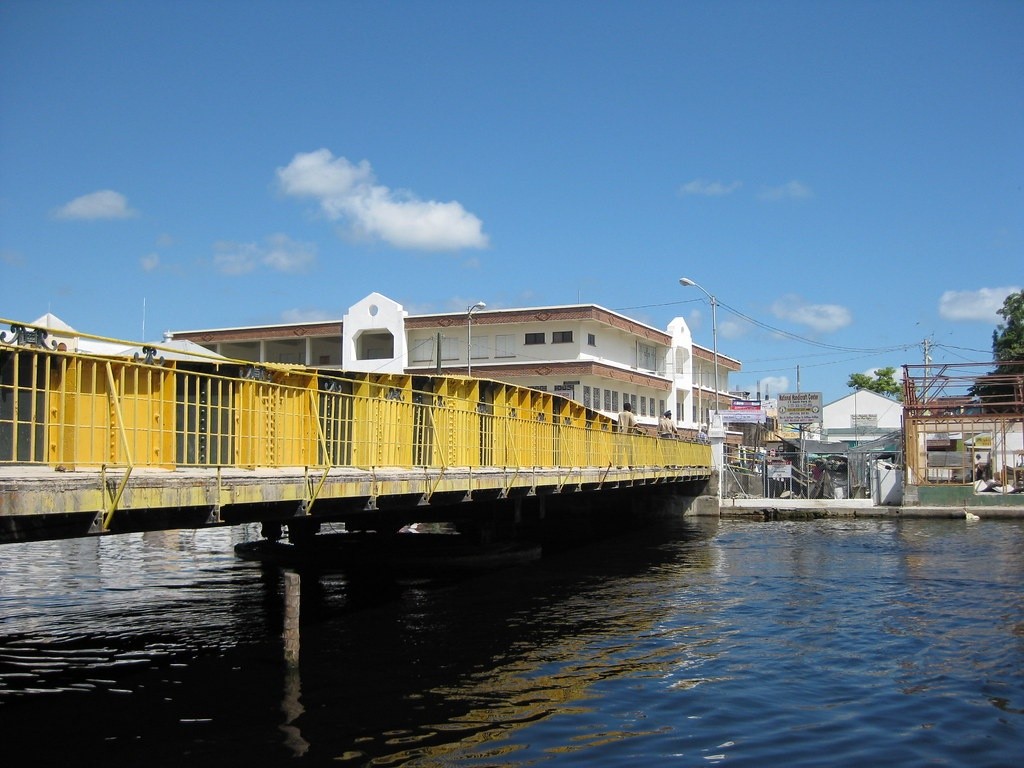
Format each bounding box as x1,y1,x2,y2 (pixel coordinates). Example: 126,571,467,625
663,410,672,415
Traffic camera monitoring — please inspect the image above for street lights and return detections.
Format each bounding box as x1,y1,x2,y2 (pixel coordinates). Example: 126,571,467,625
467,302,486,377
680,277,719,415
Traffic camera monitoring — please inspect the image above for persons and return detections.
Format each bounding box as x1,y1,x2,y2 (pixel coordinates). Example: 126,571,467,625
976,455,986,481
657,412,680,470
695,427,712,445
810,461,823,481
615,402,649,471
